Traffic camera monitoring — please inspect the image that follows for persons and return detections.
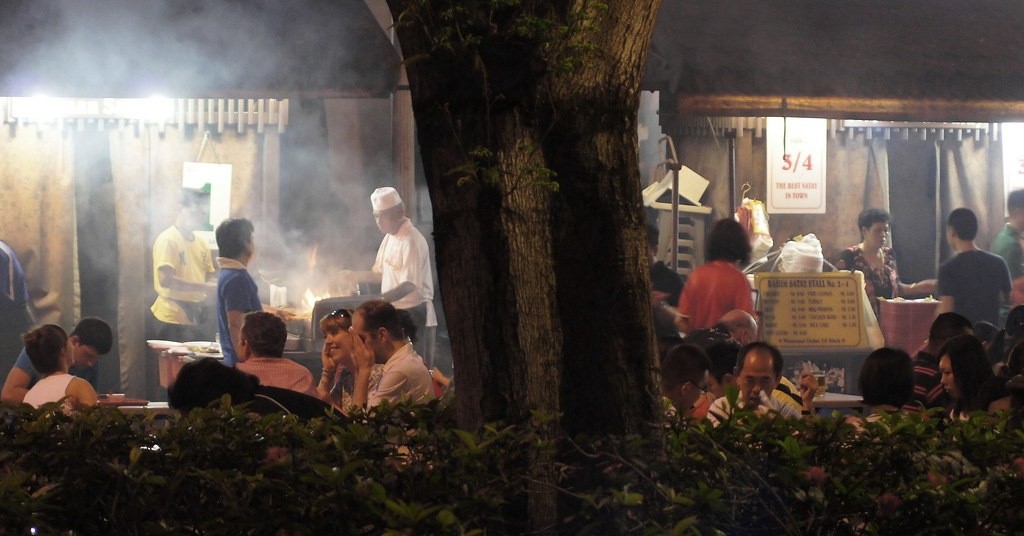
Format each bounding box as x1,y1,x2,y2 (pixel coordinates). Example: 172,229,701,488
834,207,938,323
0,240,37,393
215,216,297,367
933,188,1023,328
148,189,218,401
168,299,451,469
0,317,113,428
647,218,820,447
844,305,1024,446
339,186,438,367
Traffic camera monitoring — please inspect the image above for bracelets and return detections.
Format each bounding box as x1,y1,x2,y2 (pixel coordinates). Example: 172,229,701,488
320,368,336,378
910,283,916,296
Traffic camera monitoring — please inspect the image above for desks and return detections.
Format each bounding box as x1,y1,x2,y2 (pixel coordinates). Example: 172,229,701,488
809,394,862,414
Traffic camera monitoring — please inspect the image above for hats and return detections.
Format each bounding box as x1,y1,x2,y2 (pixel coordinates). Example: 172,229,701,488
371,187,402,211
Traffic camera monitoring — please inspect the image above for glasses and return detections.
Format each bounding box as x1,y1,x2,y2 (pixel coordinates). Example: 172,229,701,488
331,308,351,320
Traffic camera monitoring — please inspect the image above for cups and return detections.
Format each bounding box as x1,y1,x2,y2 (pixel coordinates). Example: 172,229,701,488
811,370,825,398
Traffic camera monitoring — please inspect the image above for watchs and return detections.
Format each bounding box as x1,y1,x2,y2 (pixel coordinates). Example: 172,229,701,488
801,407,815,416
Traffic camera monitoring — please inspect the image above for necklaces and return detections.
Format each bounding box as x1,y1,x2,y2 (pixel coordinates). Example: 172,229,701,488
861,243,883,270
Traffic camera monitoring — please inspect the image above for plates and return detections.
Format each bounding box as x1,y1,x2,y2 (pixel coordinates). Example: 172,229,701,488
145,340,223,358
